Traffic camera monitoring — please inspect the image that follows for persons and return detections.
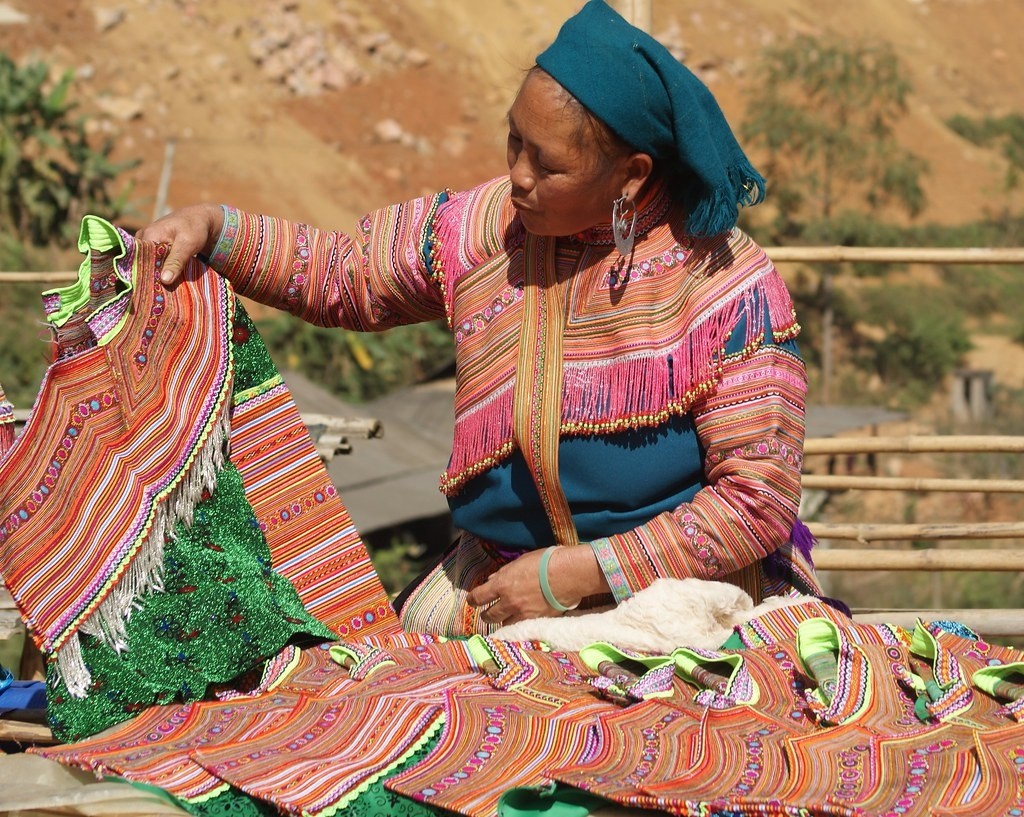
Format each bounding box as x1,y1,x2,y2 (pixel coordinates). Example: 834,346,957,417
134,0,823,637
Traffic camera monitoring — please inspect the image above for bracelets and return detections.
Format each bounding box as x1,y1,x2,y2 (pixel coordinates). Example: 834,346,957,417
539,545,582,612
